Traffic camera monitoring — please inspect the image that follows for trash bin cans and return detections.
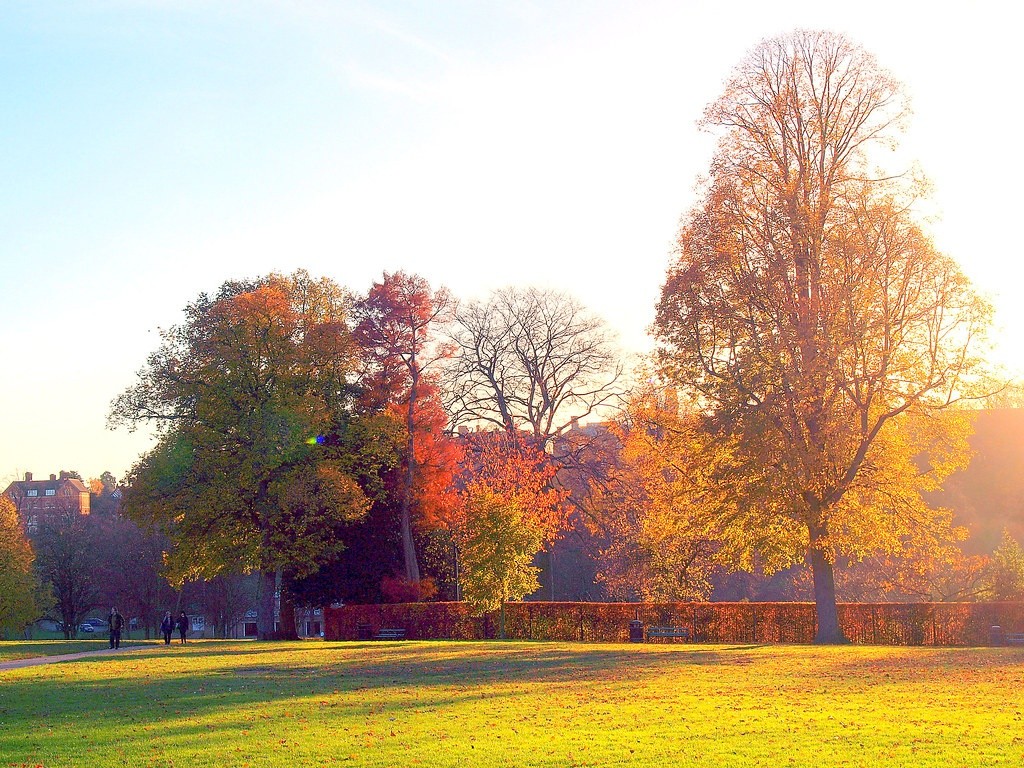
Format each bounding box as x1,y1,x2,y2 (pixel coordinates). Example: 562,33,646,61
629,620,644,643
989,626,1003,647
358,623,372,641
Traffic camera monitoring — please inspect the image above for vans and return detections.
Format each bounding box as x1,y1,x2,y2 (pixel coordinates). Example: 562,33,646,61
85,618,103,626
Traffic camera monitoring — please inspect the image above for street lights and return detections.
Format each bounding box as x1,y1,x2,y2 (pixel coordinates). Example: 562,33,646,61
543,440,555,602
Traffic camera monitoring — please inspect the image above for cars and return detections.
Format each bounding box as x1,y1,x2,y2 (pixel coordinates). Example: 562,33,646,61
56,622,70,632
80,624,94,632
121,617,143,632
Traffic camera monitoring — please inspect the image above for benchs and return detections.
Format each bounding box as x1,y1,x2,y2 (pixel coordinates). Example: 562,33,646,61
646,627,689,644
373,628,407,641
1006,633,1024,645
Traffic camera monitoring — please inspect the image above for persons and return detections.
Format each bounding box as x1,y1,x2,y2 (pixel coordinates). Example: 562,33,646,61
107,606,125,649
174,611,189,644
161,611,175,645
